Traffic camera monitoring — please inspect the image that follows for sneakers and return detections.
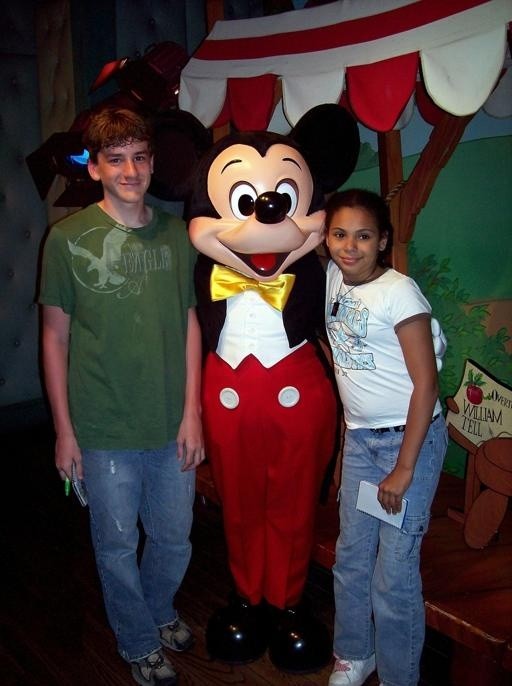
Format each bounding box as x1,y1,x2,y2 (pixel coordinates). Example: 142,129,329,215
129,647,179,685
328,650,377,686
156,618,195,653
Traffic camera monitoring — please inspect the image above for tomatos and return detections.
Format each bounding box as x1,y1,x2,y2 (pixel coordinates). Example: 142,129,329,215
465,385,483,404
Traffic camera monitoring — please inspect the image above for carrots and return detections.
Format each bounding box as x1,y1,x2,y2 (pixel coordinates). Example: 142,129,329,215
446,397,459,414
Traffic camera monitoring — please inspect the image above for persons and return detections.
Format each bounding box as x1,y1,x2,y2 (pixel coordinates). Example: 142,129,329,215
323,188,450,686
37,108,206,686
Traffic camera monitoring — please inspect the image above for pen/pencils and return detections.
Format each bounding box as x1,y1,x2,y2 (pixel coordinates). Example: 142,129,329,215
64,476,70,497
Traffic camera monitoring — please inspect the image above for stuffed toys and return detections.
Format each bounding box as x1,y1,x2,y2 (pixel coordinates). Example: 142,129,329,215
153,104,359,675
